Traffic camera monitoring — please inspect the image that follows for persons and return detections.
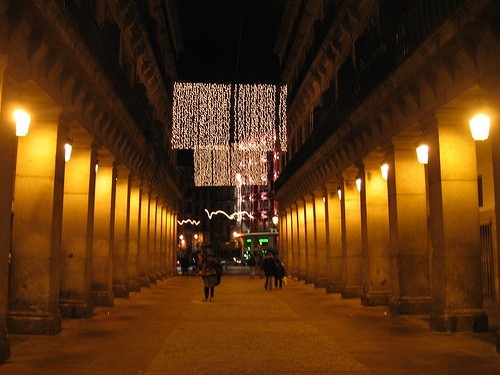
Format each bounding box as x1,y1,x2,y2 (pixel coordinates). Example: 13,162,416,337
180,254,190,276
248,254,256,279
263,252,277,290
202,254,218,302
193,253,203,276
258,256,265,279
274,258,285,289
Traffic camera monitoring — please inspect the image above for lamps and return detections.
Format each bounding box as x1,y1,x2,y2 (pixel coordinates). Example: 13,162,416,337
64,137,73,162
380,159,389,179
272,215,278,225
470,95,490,142
416,133,429,164
